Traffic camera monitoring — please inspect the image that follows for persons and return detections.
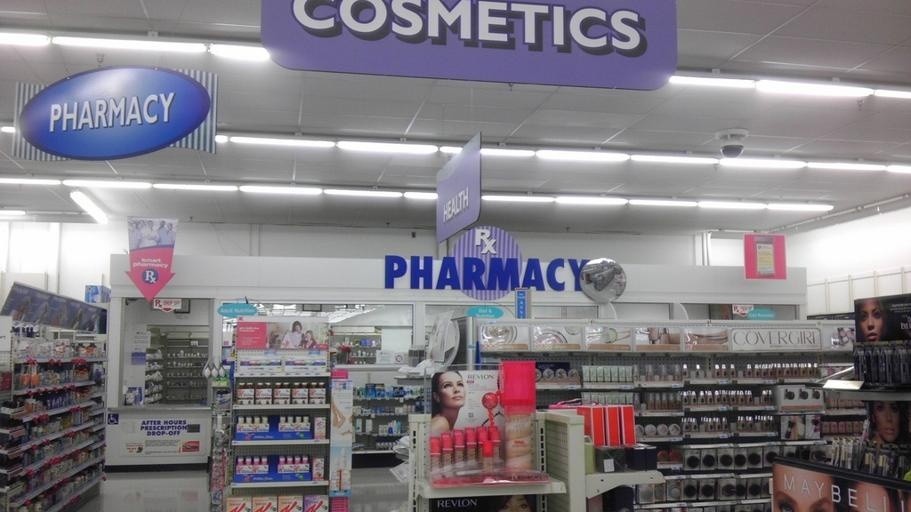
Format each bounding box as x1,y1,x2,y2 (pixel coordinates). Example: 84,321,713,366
868,400,906,447
430,371,466,437
279,320,317,350
835,478,900,512
772,463,833,512
855,298,886,342
